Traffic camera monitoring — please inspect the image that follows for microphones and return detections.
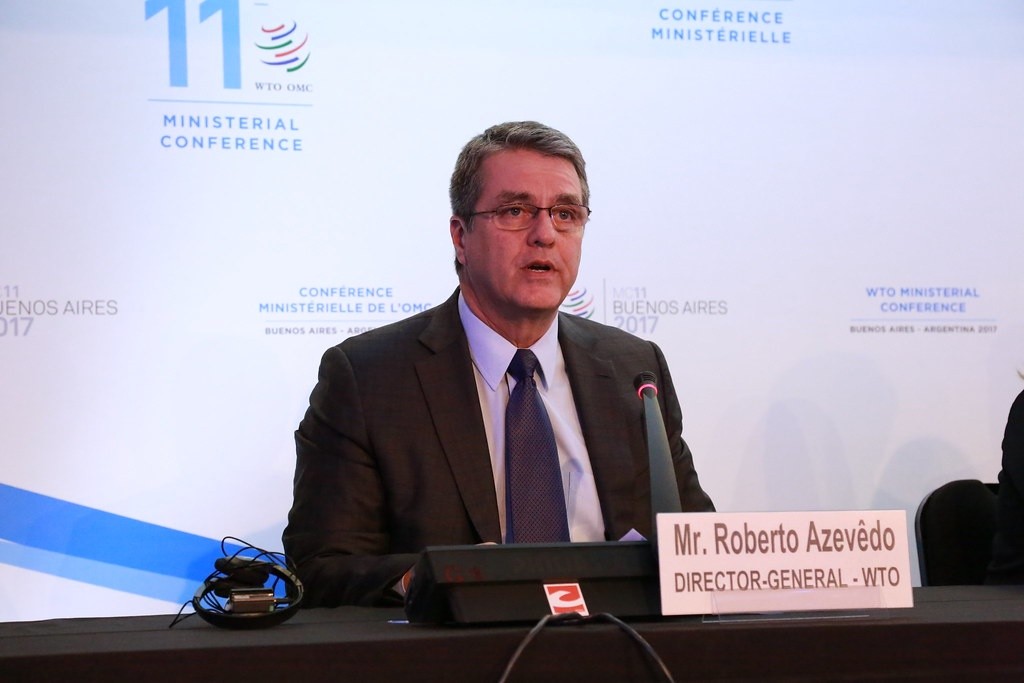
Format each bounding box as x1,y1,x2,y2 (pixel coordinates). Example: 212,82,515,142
636,371,686,535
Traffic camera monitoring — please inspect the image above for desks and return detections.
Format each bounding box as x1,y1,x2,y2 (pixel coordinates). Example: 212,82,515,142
1,581,1024,683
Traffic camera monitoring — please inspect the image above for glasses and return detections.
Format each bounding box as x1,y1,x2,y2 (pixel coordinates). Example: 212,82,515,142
465,203,591,230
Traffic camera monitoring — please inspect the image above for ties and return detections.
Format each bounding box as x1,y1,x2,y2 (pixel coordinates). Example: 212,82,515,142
504,349,571,542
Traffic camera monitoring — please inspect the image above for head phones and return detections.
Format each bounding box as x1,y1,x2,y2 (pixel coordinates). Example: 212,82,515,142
191,555,304,629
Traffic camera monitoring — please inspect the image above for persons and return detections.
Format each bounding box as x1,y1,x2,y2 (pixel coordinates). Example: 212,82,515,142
987,390,1024,584
282,121,717,606
